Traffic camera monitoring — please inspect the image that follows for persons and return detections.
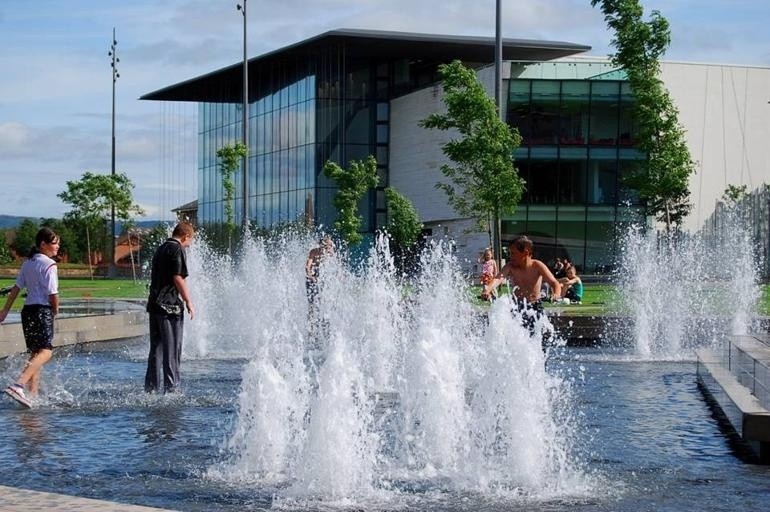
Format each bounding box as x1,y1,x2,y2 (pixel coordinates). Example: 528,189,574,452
540,255,584,305
480,236,561,335
144,221,193,393
0,227,61,409
479,247,498,297
305,238,337,343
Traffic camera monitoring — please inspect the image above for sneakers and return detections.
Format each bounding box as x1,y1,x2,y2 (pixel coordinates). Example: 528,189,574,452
5,383,33,409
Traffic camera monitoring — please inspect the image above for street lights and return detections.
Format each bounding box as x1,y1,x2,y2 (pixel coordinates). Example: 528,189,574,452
108,25,120,266
